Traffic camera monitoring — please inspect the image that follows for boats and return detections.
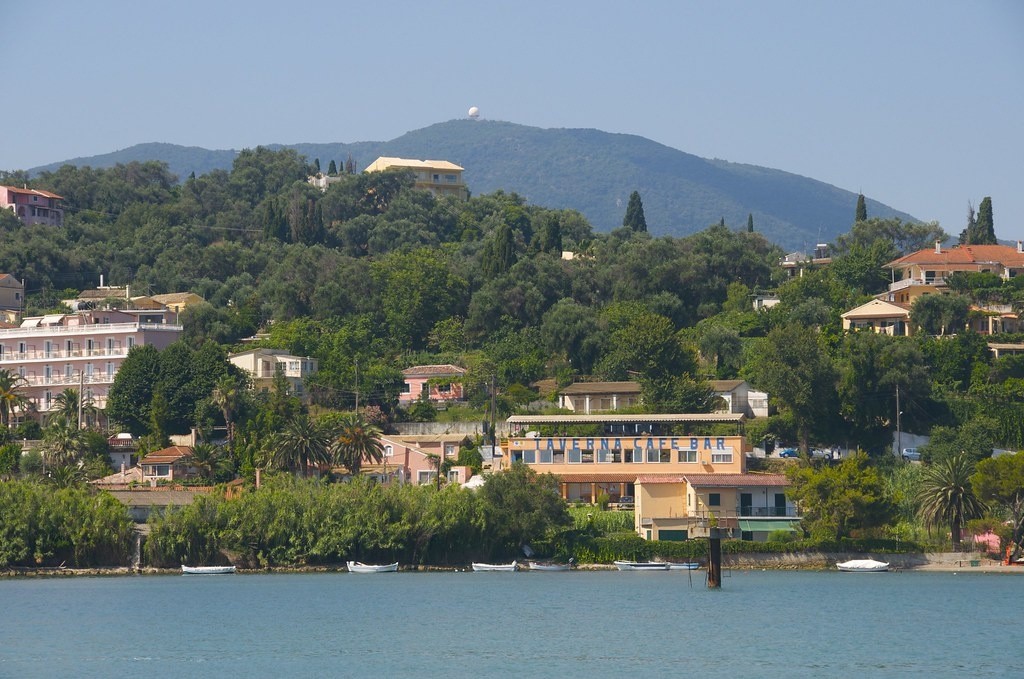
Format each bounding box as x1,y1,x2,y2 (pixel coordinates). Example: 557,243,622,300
835,560,890,571
181,563,235,573
471,560,517,573
664,560,701,571
613,559,670,570
346,560,398,573
528,557,574,572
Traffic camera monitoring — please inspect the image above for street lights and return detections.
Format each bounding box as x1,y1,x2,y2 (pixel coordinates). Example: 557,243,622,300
897,411,903,459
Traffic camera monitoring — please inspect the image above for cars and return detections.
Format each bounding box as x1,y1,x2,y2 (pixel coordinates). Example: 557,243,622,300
810,448,834,460
618,495,634,510
779,447,813,460
902,447,922,461
